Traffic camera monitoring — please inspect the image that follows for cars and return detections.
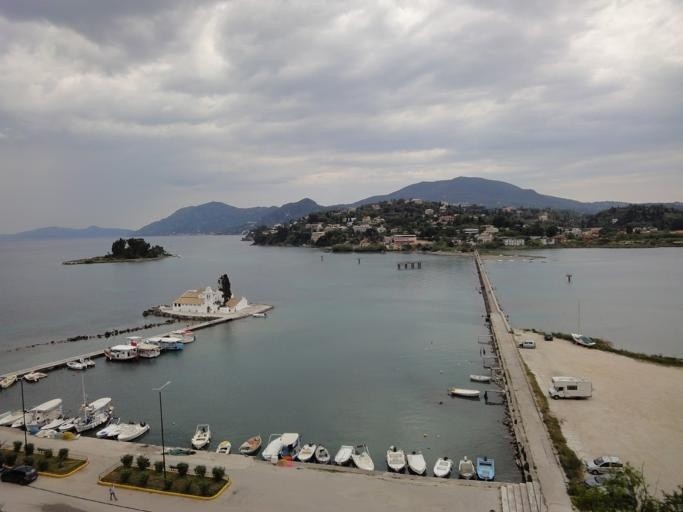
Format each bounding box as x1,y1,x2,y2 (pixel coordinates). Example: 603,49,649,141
544,331,553,341
585,456,623,488
518,339,536,348
0,464,39,485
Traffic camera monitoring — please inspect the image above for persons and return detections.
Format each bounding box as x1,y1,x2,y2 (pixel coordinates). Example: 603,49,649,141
109,482,118,501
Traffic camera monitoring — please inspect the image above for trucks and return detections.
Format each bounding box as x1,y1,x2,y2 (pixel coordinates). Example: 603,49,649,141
549,377,592,400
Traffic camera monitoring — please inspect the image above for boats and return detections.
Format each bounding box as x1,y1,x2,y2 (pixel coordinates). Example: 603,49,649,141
451,388,481,397
191,423,212,450
351,443,375,471
386,445,406,473
279,432,302,460
252,313,268,319
262,433,283,461
297,441,317,462
471,375,492,382
458,455,477,480
238,435,262,454
432,457,454,477
315,443,331,464
216,441,231,454
572,333,596,346
0,372,47,388
477,455,496,481
105,331,195,361
67,357,96,369
334,445,354,466
406,451,426,475
0,398,150,442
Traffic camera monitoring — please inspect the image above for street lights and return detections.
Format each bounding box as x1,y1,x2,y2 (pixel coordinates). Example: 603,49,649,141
11,375,34,459
152,381,173,480
